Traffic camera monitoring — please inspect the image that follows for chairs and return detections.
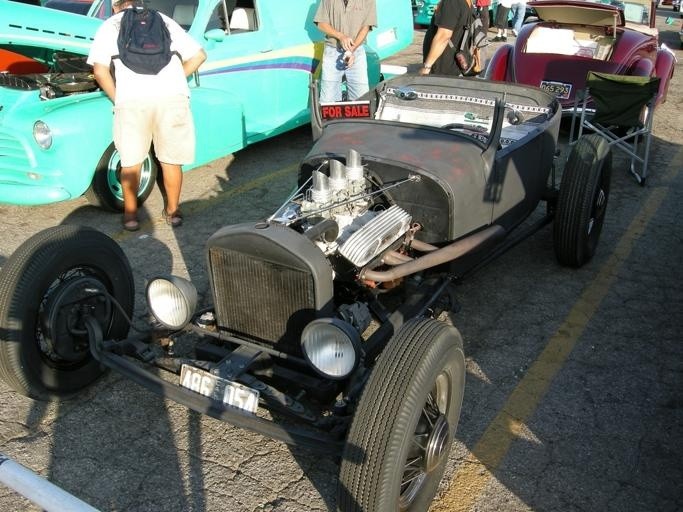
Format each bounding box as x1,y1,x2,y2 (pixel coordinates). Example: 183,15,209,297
565,70,662,186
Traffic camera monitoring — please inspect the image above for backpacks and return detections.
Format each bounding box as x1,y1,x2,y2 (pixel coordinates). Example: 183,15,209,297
454,8,489,77
117,6,172,76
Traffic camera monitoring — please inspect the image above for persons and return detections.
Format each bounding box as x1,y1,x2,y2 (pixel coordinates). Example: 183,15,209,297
509,0,527,39
85,1,209,232
476,0,491,35
488,0,512,42
418,0,474,77
312,0,378,102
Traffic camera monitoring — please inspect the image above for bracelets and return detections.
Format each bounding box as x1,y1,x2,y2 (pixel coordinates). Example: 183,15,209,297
423,63,431,69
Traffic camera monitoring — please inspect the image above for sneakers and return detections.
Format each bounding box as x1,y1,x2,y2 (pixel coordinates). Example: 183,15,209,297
489,36,507,42
122,218,139,231
164,209,183,227
